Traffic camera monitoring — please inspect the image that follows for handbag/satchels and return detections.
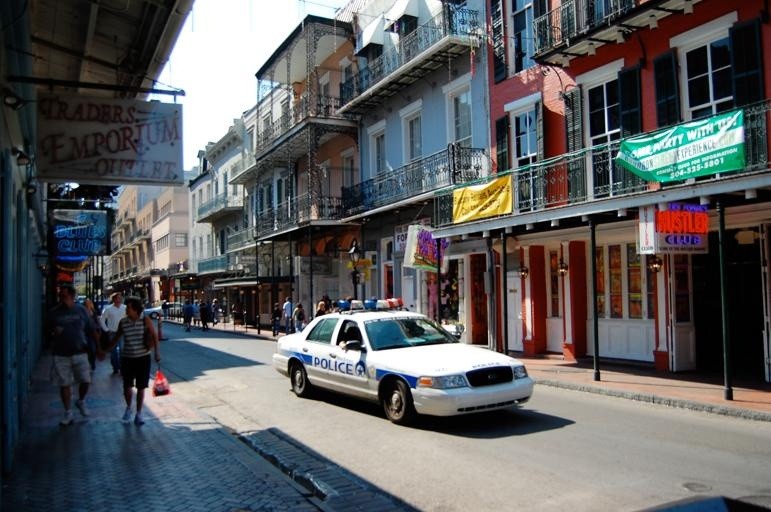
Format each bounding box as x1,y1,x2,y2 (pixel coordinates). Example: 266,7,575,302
142,327,155,351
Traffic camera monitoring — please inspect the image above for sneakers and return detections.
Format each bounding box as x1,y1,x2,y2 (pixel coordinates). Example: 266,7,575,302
185,326,209,332
111,370,120,375
273,334,276,336
90,362,97,370
135,413,145,425
61,410,75,424
122,407,134,422
277,332,279,335
75,400,90,416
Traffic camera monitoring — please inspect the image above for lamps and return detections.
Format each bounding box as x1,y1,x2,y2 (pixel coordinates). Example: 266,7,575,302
21,181,36,193
561,0,694,69
11,145,31,165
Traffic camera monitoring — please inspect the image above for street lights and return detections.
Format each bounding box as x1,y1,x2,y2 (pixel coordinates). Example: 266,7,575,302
348,239,364,300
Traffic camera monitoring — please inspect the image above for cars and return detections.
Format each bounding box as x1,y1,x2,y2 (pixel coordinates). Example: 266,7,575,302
271,296,535,424
78,295,111,313
144,302,184,320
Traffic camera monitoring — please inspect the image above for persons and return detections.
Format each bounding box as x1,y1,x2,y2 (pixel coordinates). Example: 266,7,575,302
44,285,103,425
100,296,160,425
99,292,127,374
271,296,354,338
182,296,221,332
84,299,98,369
161,300,169,319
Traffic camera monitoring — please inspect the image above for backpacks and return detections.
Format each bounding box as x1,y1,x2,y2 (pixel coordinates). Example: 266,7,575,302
297,308,304,321
